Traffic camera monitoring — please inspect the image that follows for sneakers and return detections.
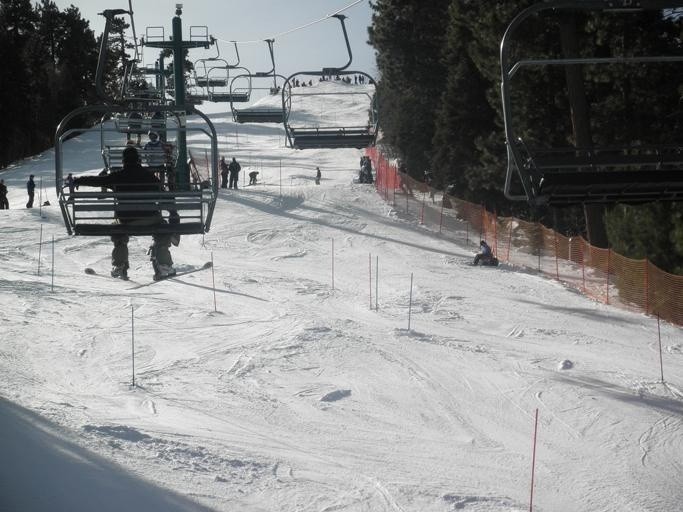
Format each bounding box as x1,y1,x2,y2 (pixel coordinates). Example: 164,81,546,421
150,257,176,277
110,265,128,279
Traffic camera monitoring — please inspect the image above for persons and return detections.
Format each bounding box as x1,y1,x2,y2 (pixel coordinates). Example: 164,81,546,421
97,168,107,200
150,111,166,142
25,174,35,208
125,111,142,145
0,179,8,209
64,173,74,203
143,132,166,191
472,240,491,265
228,157,241,190
248,171,259,185
359,157,362,167
361,156,366,167
362,156,371,172
267,73,364,96
314,166,321,185
218,156,229,188
61,147,181,282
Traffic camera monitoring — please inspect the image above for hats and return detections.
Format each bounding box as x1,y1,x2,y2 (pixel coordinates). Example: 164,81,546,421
121,148,139,162
